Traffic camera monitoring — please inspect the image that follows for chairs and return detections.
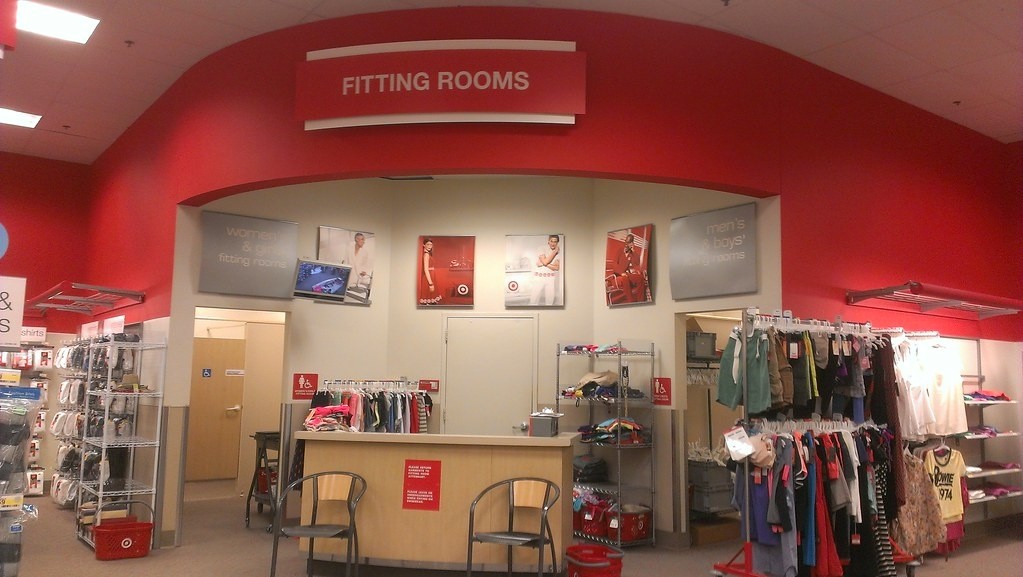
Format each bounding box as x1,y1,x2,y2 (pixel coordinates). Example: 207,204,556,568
466,477,558,577
270,472,367,577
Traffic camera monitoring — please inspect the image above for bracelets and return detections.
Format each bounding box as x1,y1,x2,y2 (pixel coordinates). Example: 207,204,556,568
429,284,434,286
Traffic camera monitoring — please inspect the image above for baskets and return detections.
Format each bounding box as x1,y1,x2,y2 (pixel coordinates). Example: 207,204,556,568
572,502,582,530
604,502,653,542
563,543,625,577
93,499,155,560
580,500,620,536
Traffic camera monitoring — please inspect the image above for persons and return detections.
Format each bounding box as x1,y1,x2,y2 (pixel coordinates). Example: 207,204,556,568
344,233,366,288
613,234,645,303
531,235,560,305
420,238,442,305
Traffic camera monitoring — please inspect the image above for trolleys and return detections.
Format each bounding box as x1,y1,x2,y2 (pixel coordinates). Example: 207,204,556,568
244,431,280,534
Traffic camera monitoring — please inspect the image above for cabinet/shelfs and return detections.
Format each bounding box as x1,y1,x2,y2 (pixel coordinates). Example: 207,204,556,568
245,430,281,533
687,458,738,515
554,342,656,550
963,400,1023,504
0,335,168,552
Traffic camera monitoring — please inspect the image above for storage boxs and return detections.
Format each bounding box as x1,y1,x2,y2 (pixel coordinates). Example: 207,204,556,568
685,332,716,358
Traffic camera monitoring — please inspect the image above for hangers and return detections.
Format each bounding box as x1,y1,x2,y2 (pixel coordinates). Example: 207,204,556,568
316,379,427,400
758,417,951,459
735,314,951,350
